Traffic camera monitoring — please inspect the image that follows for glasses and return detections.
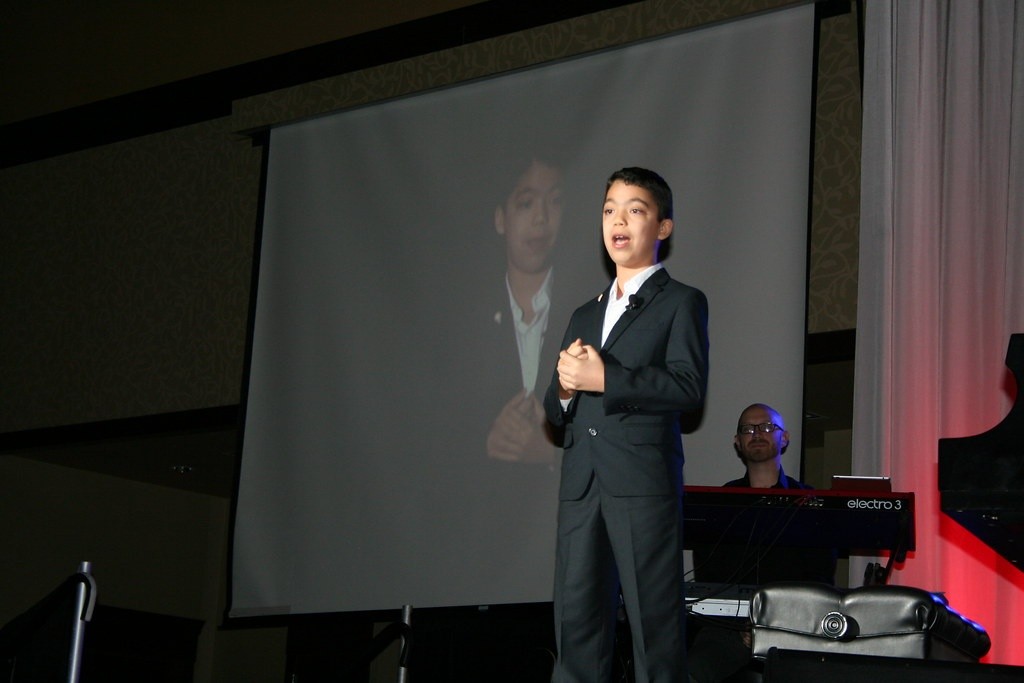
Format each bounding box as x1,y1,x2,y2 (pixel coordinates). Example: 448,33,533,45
738,423,784,434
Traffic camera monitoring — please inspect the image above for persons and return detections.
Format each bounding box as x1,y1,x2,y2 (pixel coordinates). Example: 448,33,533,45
430,152,572,604
544,167,703,682
687,402,838,683
